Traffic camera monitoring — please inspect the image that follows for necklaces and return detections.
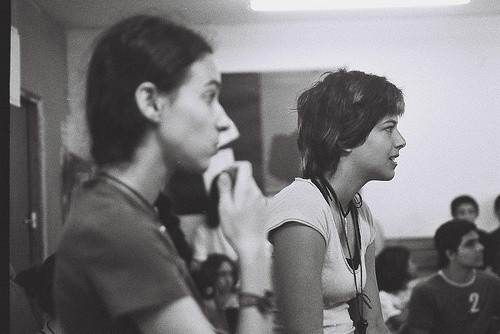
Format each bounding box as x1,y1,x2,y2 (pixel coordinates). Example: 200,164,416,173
323,177,373,334
93,170,155,211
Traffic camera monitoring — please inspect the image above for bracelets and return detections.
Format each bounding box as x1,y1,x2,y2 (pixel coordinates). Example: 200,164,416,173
238,290,276,314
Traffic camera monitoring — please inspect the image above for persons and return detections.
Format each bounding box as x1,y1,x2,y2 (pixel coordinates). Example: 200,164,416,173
52,15,277,334
374,195,500,334
184,205,240,334
266,70,407,334
